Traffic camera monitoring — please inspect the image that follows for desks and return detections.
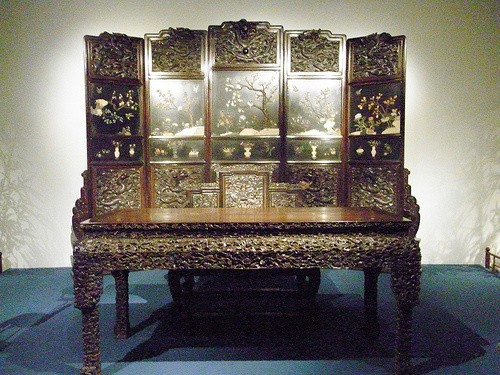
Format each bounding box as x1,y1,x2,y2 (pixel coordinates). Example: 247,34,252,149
69,165,421,373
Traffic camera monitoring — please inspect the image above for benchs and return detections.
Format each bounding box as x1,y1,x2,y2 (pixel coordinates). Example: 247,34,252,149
167,268,322,318
